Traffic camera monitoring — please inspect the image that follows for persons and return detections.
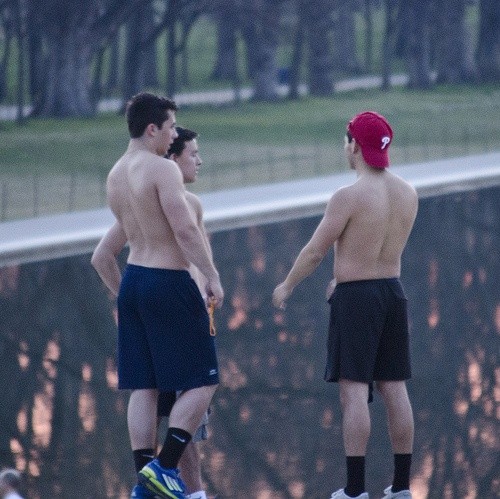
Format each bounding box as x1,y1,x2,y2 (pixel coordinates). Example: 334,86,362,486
106,91,224,499
92,127,214,498
0,468,24,499
272,110,418,498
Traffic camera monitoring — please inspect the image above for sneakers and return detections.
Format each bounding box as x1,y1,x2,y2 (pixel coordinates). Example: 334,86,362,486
331,488,369,499
382,484,412,499
138,458,191,499
131,484,163,499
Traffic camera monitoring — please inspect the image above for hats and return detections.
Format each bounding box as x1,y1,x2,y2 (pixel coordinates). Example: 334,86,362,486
346,111,393,168
